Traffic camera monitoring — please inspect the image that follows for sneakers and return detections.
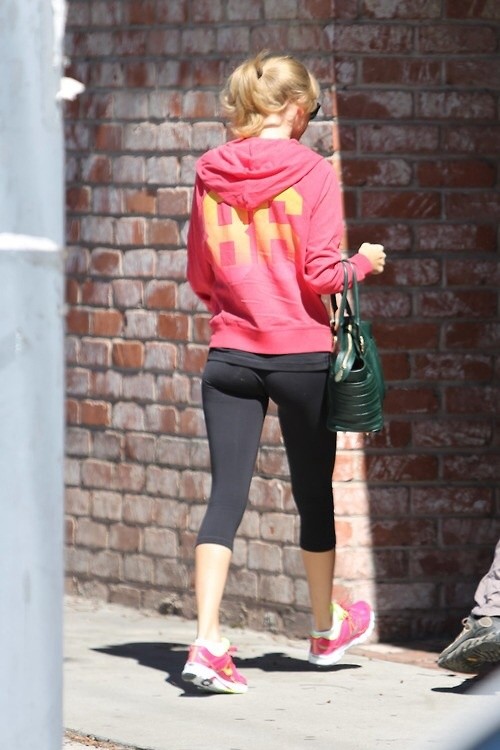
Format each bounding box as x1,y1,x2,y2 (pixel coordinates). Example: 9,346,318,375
436,615,500,673
310,601,374,665
182,636,247,693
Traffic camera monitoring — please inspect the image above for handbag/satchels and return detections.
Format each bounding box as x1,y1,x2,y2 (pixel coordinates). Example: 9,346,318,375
329,259,385,433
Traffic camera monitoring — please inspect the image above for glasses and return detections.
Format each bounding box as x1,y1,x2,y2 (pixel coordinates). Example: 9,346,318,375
310,101,321,120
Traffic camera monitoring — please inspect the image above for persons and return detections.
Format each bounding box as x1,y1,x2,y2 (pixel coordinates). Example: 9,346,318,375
181,48,386,694
434,539,500,675
